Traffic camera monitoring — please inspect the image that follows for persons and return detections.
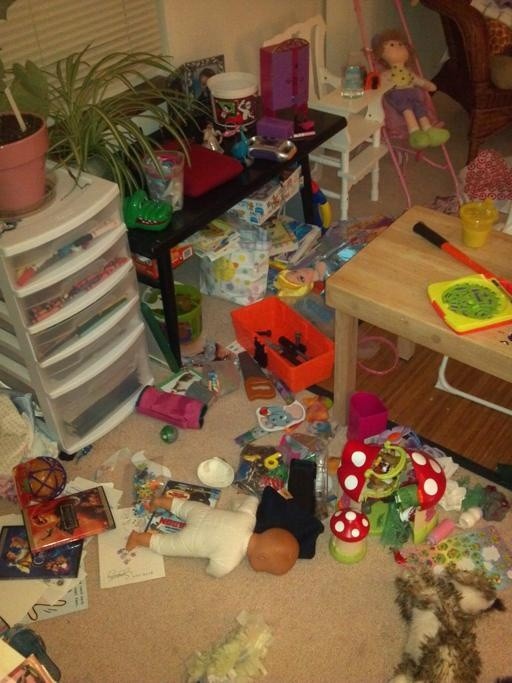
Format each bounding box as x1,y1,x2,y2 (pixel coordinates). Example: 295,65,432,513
372,30,451,151
124,493,299,577
196,68,217,101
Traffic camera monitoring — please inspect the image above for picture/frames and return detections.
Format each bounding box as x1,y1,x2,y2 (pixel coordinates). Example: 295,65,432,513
178,52,226,119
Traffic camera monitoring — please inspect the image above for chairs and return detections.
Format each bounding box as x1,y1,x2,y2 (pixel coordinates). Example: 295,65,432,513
419,0,511,167
264,11,385,222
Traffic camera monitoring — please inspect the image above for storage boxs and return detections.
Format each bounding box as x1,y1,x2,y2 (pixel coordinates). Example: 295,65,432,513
229,293,336,398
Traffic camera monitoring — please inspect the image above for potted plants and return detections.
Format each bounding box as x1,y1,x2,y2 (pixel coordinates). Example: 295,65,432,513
15,43,216,203
0,58,49,213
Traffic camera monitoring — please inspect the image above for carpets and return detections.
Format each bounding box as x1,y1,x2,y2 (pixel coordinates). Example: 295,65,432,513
0,383,512,682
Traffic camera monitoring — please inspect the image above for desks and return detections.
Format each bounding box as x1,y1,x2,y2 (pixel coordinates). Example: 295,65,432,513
323,199,512,439
83,91,350,383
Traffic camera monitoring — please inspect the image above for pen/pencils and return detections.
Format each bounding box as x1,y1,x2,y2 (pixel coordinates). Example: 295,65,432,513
16,245,71,287
74,220,123,247
291,131,316,138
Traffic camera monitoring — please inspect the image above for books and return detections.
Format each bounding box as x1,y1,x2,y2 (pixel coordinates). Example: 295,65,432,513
1,526,84,579
22,486,117,552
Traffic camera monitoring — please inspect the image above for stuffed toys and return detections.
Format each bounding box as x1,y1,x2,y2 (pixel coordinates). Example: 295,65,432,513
388,565,505,683
185,611,275,683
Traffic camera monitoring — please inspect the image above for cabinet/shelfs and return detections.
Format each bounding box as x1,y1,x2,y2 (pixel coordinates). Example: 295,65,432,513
0,159,156,460
